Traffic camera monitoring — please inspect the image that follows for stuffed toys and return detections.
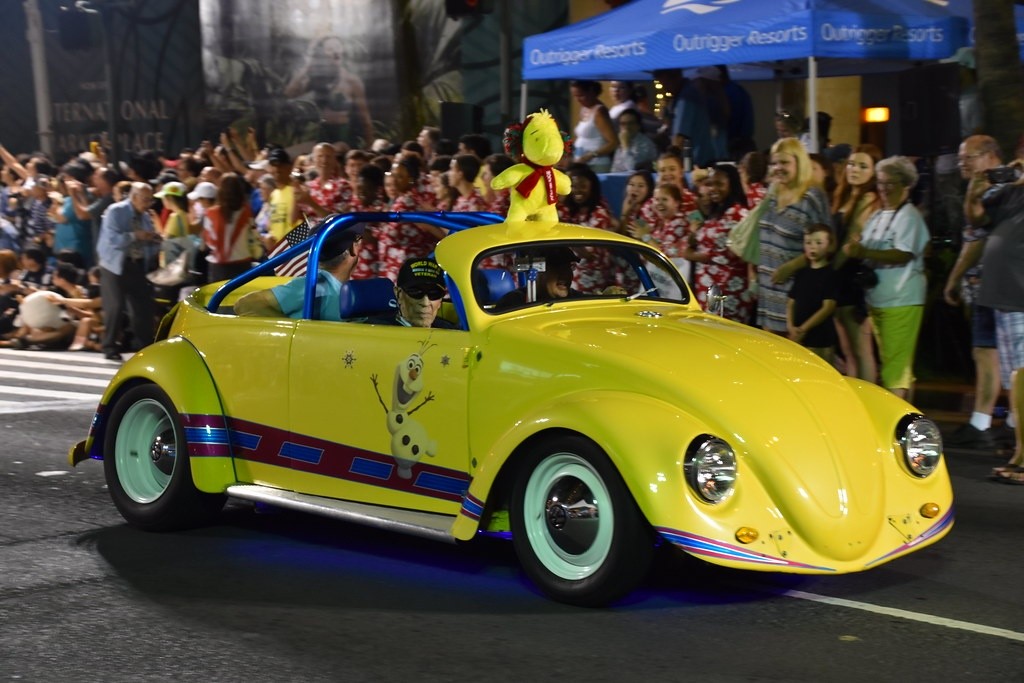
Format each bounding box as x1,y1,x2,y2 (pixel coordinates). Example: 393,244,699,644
490,107,571,226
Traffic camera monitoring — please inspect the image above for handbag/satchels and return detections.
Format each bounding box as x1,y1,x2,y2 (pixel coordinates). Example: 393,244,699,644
165,234,202,270
726,202,764,262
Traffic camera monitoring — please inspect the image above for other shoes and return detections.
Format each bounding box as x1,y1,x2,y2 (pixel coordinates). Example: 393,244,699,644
942,422,1016,450
993,463,1024,483
107,353,121,360
11,337,30,349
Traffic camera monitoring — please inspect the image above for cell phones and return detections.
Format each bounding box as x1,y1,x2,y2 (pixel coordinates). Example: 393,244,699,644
983,166,1019,185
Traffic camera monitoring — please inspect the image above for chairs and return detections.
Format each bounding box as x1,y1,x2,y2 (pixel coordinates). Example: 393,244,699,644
340,277,398,319
473,269,515,306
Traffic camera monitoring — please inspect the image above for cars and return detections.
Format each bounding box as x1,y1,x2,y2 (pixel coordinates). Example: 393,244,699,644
70,208,957,609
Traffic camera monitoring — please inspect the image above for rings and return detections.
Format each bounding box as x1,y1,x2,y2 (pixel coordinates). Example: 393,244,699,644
619,289,625,293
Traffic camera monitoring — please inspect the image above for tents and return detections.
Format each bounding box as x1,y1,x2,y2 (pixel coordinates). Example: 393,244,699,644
523,0,1024,156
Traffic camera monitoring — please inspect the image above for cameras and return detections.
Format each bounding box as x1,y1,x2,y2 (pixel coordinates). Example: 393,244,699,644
292,171,318,182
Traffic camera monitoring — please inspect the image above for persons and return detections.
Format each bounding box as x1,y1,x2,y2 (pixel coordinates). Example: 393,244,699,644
283,37,374,161
0,66,1024,486
426,0,508,147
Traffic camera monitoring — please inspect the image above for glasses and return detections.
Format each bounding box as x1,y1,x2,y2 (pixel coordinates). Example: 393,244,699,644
547,262,577,271
953,151,989,163
400,284,445,300
138,193,155,204
876,180,903,187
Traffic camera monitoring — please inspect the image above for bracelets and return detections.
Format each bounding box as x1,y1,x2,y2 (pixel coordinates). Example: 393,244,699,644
748,277,760,284
619,214,628,218
640,233,652,243
588,149,599,156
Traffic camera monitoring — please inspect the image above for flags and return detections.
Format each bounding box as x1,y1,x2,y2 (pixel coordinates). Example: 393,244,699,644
267,219,314,277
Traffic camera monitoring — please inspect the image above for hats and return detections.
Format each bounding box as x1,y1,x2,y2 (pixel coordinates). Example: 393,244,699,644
186,182,217,201
319,221,366,260
248,160,276,172
269,149,289,163
398,257,447,290
530,245,581,263
153,182,185,199
